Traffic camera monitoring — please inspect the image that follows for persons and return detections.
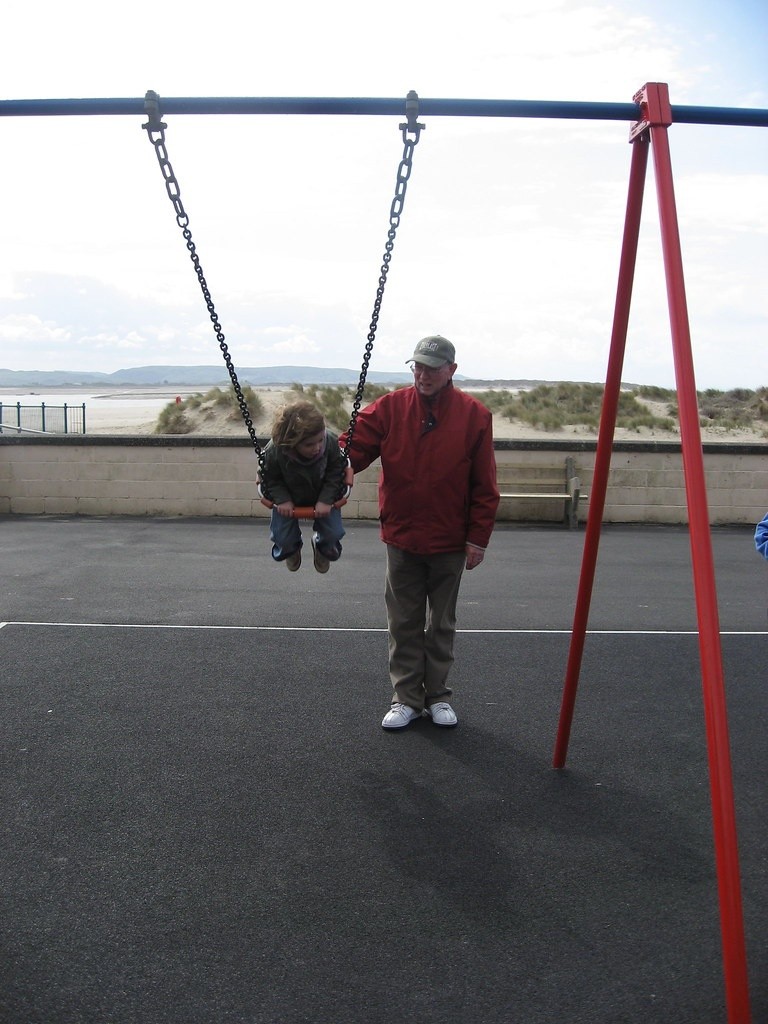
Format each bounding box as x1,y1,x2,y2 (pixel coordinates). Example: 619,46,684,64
264,401,346,573
339,334,499,729
754,512,768,559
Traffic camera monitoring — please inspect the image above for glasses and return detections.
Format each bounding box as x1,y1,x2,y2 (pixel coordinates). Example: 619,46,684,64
410,362,449,375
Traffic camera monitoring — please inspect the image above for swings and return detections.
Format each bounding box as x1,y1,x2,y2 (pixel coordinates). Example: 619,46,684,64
146,123,420,519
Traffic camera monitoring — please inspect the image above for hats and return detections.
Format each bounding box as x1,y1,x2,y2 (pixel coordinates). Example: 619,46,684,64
404,334,455,367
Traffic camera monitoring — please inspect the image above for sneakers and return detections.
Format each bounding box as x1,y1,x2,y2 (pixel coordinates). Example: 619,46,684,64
286,535,301,571
382,704,424,727
424,702,458,725
312,534,330,573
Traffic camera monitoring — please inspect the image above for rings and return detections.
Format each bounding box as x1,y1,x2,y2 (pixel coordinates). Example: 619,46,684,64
480,560,481,562
